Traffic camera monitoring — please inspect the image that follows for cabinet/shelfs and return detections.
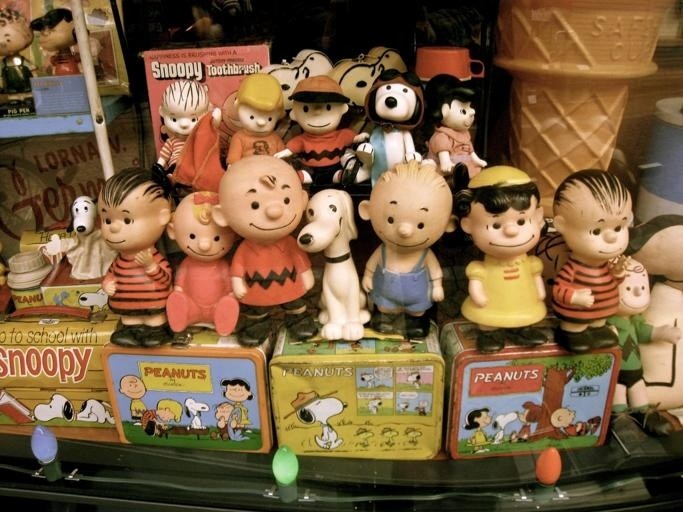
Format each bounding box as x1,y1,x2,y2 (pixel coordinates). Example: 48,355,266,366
1,0,132,181
